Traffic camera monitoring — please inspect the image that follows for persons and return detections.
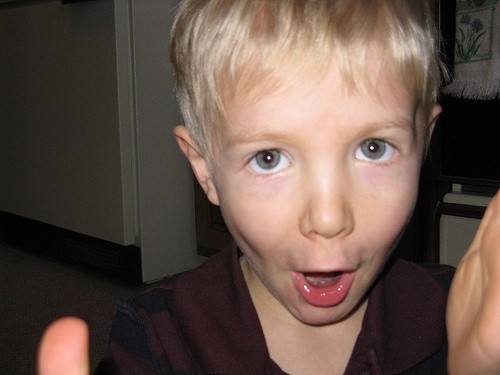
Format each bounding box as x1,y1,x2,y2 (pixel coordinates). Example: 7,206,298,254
37,0,500,375
